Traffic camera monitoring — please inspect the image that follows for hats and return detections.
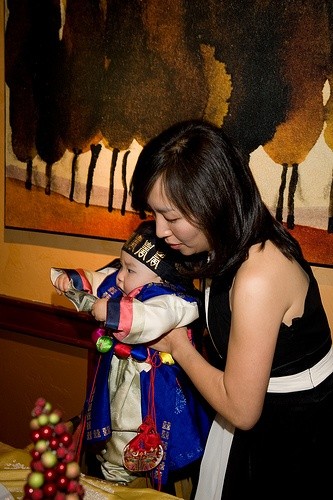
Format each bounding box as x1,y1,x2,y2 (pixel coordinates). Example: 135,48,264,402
120,220,195,289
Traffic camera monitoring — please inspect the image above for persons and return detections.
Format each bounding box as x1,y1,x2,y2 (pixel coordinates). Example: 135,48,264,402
130,117,333,499
49,218,211,487
